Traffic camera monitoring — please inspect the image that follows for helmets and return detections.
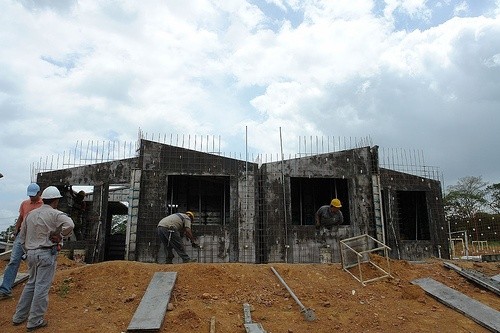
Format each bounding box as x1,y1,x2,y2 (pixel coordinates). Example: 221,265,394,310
42,186,63,199
331,199,342,207
79,191,85,197
186,212,194,221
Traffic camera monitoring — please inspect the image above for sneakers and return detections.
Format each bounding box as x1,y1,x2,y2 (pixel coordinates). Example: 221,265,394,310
183,258,197,263
12,314,29,325
0,292,12,298
26,319,48,332
166,261,173,264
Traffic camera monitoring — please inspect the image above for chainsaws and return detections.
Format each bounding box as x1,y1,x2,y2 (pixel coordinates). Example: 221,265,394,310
192,242,203,249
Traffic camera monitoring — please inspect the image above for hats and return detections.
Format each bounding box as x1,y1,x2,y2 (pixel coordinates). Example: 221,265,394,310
27,183,40,196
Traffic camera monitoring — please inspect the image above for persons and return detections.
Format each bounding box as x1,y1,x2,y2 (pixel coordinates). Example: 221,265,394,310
13,185,73,330
157,210,199,263
70,185,86,240
315,198,344,225
0,183,41,299
